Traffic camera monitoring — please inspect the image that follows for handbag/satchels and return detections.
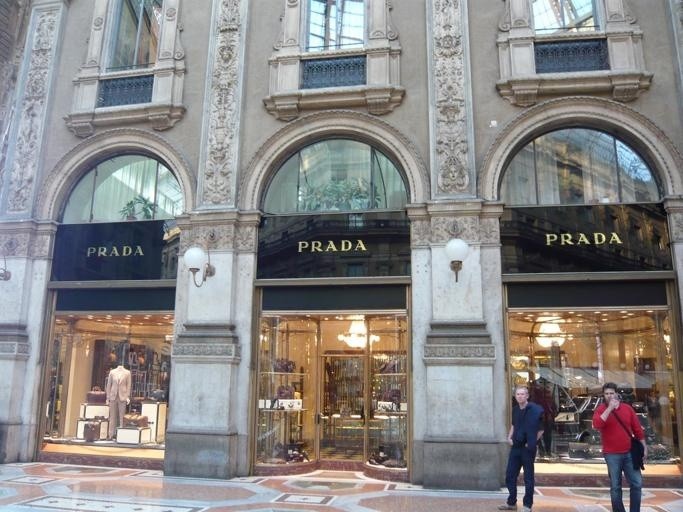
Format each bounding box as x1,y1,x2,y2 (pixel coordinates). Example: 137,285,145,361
283,441,305,463
123,411,148,429
275,357,295,372
631,434,645,471
568,441,590,459
86,385,107,404
276,383,295,399
371,359,402,464
84,423,101,442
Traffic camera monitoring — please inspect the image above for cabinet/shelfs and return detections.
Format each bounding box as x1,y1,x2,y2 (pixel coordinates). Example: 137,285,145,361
258,369,309,467
371,370,409,469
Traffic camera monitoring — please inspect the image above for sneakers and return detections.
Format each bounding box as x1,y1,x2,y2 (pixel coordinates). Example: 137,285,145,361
496,501,517,510
520,504,532,512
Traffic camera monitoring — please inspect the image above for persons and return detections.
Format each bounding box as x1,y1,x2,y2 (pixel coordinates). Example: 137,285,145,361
495,384,545,511
590,377,648,512
105,365,133,440
